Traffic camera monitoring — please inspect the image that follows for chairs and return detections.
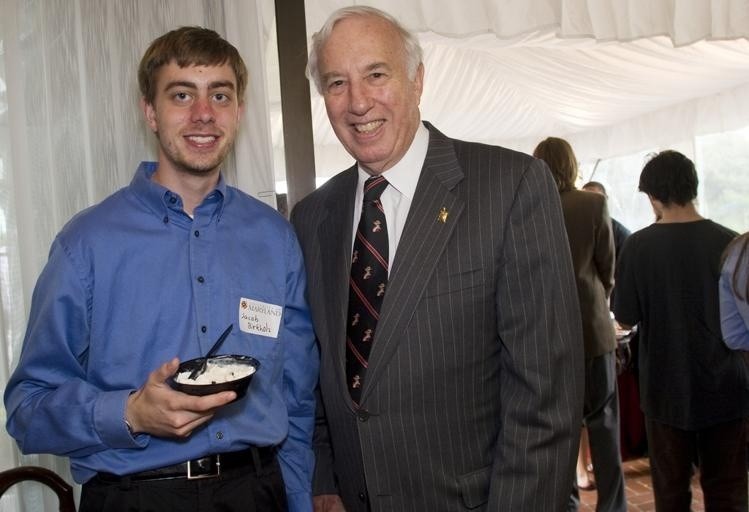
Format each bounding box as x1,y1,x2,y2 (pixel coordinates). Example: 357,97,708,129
1,466,75,511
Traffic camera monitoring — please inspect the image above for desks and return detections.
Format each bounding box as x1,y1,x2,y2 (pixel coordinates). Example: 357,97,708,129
612,326,644,461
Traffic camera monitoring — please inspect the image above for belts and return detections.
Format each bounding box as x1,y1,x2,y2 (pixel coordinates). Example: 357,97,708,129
83,445,279,485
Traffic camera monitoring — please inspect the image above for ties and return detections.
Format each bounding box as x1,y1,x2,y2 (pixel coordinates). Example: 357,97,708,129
344,176,390,407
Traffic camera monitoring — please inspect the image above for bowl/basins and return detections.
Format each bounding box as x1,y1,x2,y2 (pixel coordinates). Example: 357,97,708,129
164,355,261,406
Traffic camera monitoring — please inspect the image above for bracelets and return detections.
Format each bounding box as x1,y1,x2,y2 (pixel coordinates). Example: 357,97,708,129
125,420,141,439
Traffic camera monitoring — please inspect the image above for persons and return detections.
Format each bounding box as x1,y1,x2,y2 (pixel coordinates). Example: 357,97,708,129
581,178,639,311
0,22,322,511
533,135,633,511
573,420,595,491
611,150,748,511
714,226,749,352
289,5,586,511
